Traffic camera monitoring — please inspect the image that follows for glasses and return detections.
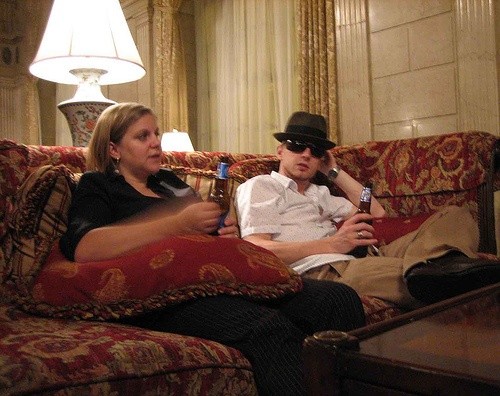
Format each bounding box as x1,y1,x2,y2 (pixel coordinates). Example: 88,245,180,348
286,139,325,159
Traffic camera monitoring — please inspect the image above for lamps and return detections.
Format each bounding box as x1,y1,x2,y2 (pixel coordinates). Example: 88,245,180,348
28,0,148,148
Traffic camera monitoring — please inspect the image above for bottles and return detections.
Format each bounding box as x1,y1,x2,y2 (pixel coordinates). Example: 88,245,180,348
207,157,230,237
350,182,373,258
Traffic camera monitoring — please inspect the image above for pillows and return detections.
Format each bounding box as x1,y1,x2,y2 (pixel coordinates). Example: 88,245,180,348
19,227,302,321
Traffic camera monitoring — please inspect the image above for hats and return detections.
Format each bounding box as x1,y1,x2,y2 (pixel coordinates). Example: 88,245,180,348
273,112,337,150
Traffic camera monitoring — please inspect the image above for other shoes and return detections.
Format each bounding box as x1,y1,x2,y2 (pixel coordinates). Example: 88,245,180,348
408,252,500,304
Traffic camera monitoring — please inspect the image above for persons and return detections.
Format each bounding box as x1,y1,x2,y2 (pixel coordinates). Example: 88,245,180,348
62,102,365,396
235,111,500,306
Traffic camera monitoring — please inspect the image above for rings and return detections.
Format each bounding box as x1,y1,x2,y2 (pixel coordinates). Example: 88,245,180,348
358,231,365,239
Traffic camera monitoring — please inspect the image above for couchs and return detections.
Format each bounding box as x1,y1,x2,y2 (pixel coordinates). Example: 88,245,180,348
0,131,500,396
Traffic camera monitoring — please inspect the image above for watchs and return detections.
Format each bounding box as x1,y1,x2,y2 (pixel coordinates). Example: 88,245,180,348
327,165,341,180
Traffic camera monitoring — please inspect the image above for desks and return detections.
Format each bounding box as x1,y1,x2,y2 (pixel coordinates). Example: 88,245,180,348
304,280,500,396
